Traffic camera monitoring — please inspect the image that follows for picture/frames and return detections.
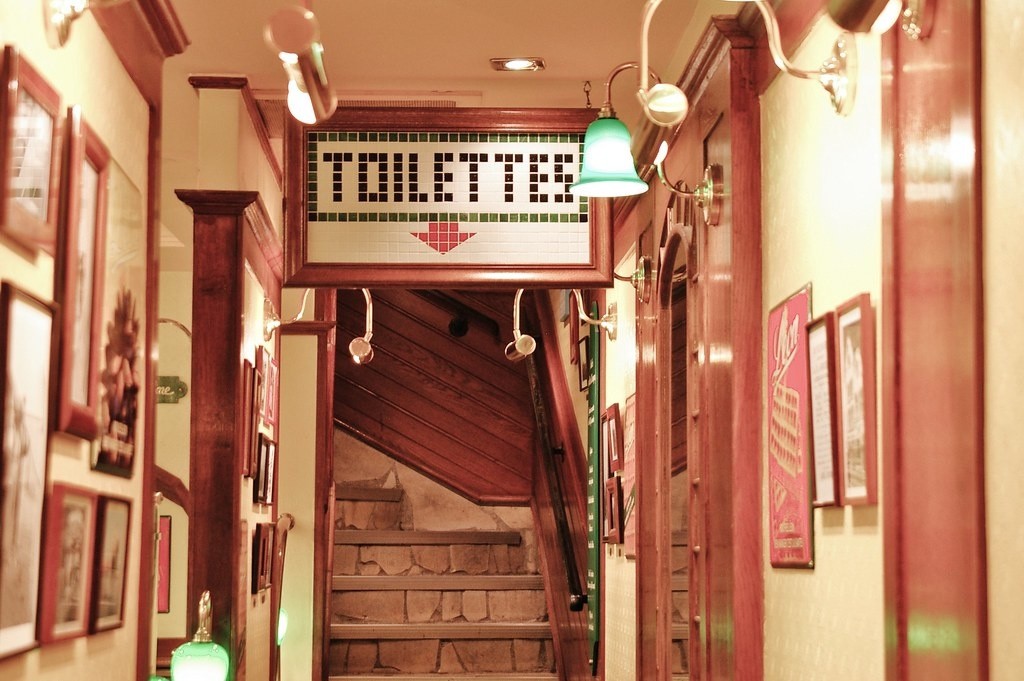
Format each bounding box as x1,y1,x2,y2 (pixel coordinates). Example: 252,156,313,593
242,344,279,595
56,102,109,441
0,278,60,662
578,336,587,393
91,491,132,634
559,286,590,366
605,476,624,545
805,312,843,509
1,44,62,256
599,410,613,543
605,403,624,473
39,479,99,643
837,294,879,507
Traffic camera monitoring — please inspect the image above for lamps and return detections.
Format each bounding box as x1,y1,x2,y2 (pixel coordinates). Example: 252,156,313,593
263,286,375,366
569,60,723,227
170,591,229,681
825,1,936,42
264,0,338,124
635,1,857,126
505,287,618,361
614,255,652,305
